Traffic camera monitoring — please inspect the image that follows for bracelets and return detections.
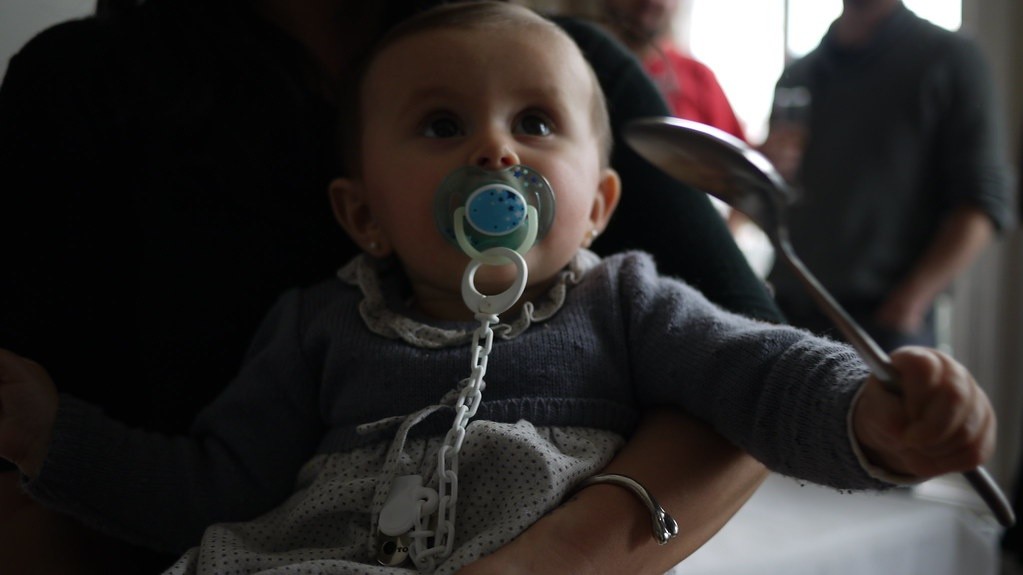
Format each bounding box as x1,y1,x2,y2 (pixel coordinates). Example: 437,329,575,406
567,472,680,548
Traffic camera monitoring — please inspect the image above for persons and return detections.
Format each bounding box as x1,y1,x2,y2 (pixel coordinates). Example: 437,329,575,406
596,0,1013,356
0,0,788,575
0,0,998,575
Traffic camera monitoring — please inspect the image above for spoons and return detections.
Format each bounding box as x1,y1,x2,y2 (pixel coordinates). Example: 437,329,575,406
624,117,1015,528
